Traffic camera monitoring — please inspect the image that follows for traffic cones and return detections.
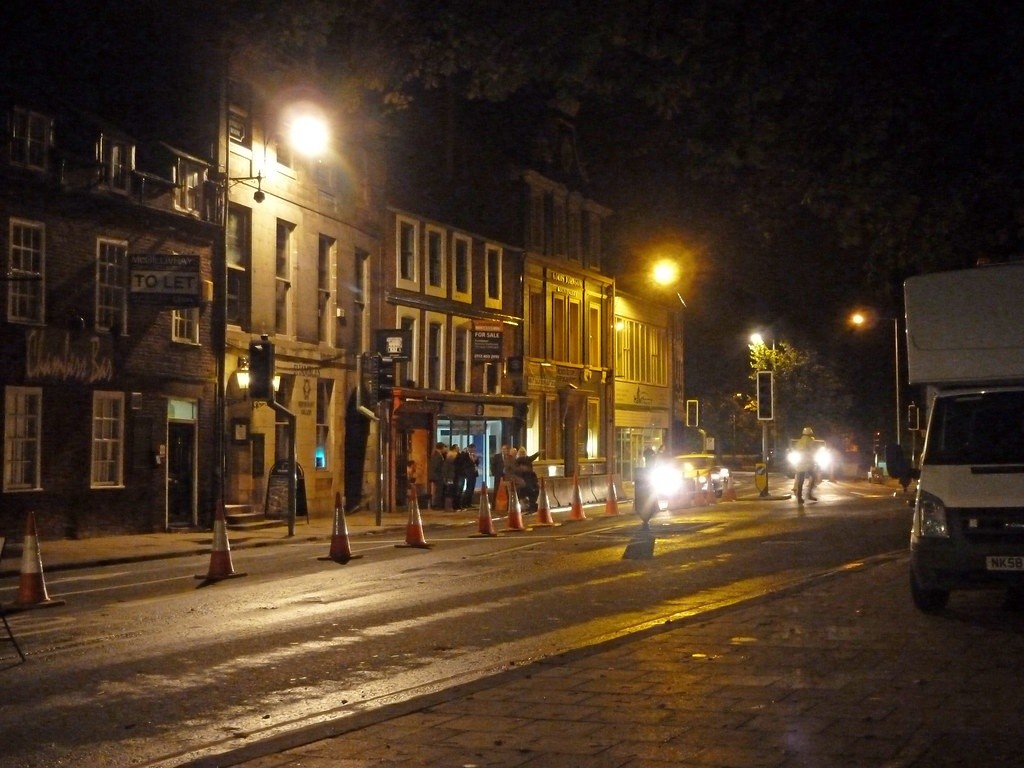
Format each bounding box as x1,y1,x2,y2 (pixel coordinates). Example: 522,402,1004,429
499,480,533,532
470,481,505,538
667,469,737,510
193,499,248,580
562,472,592,521
317,491,364,561
5,511,67,611
599,473,625,517
528,477,562,528
392,483,435,548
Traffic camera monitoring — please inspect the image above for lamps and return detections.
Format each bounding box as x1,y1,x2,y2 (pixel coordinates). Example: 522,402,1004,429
236,357,250,389
229,170,265,203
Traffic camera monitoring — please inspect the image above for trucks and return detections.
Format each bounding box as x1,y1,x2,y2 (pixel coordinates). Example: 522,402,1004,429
882,260,1024,622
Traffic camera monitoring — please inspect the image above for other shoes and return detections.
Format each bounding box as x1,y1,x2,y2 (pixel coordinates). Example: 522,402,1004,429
798,499,804,503
808,497,817,501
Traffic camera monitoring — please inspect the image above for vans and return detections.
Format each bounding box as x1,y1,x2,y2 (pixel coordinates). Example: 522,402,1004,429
784,438,831,479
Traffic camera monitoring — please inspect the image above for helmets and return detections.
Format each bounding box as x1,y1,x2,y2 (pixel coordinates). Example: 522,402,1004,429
802,427,813,435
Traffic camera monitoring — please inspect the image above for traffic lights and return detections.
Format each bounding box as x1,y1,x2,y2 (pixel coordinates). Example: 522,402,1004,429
874,431,880,452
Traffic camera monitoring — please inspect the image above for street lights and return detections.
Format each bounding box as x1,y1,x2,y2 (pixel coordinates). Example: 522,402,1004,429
852,313,901,447
652,259,681,458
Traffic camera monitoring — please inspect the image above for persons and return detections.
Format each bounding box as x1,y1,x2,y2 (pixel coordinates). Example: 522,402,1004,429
795,427,818,504
430,442,546,512
